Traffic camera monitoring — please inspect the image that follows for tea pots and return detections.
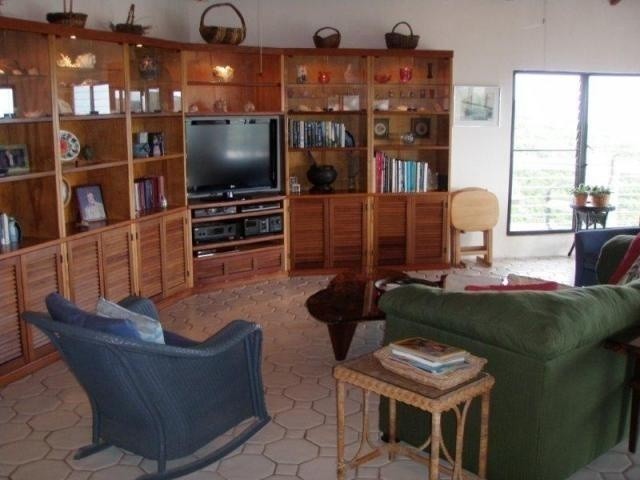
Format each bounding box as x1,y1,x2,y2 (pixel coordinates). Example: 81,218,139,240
8,218,22,244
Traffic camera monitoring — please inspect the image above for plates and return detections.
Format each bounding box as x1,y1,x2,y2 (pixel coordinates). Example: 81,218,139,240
59,130,80,162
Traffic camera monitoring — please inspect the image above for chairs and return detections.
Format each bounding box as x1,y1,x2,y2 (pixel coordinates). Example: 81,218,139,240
21,295,272,480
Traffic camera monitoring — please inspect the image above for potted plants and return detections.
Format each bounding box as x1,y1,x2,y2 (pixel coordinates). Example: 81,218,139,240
591,185,611,207
570,185,588,205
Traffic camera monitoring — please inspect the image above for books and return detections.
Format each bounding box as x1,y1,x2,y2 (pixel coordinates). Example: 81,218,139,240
372,147,439,195
372,331,488,390
286,115,349,149
134,173,170,214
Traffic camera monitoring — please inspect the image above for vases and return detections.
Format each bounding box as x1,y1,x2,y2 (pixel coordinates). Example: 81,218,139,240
317,70,330,83
138,52,160,82
399,66,412,83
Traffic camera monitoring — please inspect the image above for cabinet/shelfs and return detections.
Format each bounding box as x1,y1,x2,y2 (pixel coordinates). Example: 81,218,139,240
281,48,373,278
122,36,190,304
51,21,133,317
1,17,63,389
367,49,454,272
184,44,288,291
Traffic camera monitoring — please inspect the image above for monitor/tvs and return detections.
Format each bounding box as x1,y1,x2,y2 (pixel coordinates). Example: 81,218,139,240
184,115,282,202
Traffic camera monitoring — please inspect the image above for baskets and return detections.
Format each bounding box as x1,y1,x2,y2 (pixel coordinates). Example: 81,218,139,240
47,0,88,27
385,22,419,49
109,3,151,34
199,3,246,45
313,27,341,49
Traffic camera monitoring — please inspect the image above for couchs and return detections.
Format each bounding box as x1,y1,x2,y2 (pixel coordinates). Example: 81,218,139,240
379,231,640,478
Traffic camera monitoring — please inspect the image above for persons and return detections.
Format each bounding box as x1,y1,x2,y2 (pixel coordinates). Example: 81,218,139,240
83,190,105,220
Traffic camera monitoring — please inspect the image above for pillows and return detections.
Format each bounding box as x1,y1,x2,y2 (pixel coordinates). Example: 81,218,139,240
461,237,640,293
44,292,141,339
95,296,166,344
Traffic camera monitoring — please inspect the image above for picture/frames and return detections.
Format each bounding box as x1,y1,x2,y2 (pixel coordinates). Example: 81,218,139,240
1,80,181,118
453,83,502,128
1,143,32,174
73,184,108,224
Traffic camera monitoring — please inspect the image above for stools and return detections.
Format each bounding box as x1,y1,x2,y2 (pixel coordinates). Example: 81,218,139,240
450,186,500,268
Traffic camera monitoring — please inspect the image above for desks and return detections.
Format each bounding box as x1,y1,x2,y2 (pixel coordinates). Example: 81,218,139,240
333,345,495,480
601,324,640,459
568,205,615,257
